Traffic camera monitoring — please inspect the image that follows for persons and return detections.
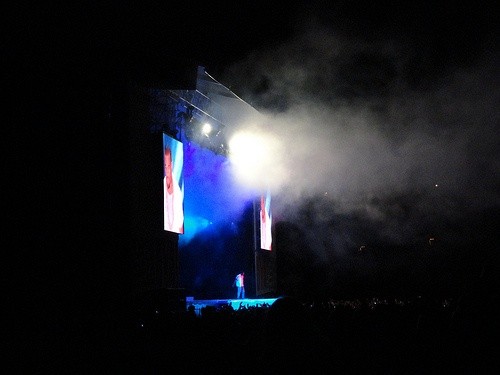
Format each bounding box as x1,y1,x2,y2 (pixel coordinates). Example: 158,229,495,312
232,272,246,298
257,195,272,249
165,149,183,233
